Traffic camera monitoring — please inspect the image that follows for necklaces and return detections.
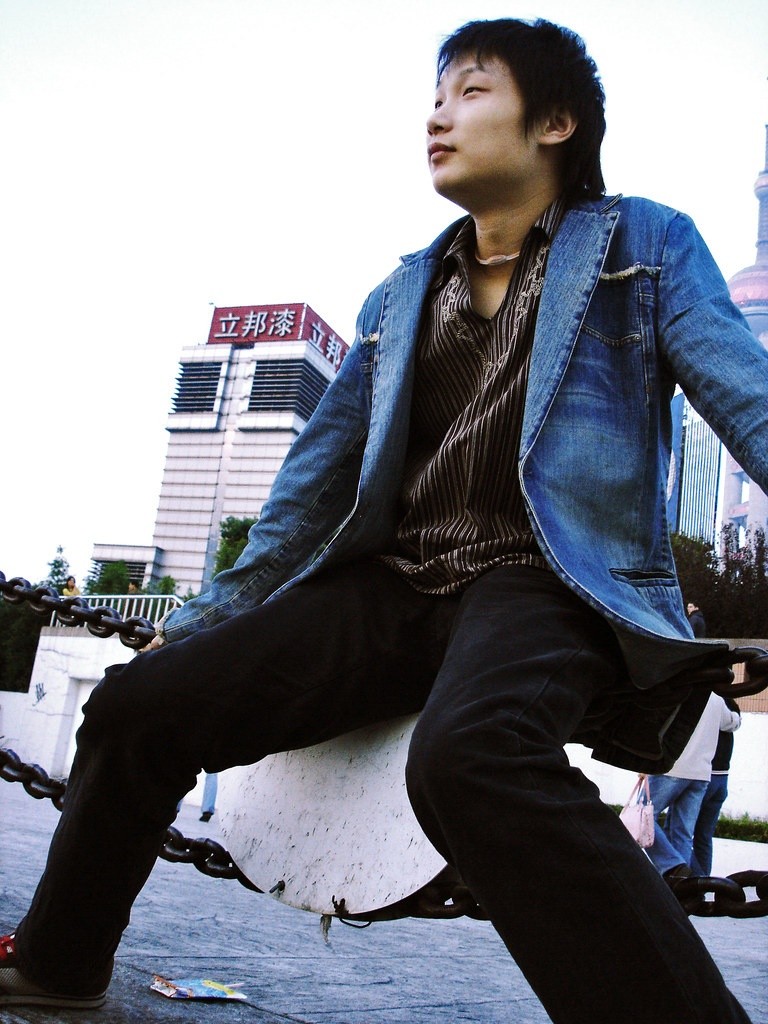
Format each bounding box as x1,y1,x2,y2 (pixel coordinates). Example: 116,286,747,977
475,251,521,266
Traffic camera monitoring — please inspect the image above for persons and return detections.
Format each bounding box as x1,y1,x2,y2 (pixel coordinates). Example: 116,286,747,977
0,19,768,1024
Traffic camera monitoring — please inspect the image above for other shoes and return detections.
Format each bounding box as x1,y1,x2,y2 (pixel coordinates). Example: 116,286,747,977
665,864,692,892
0,931,114,1010
199,812,213,823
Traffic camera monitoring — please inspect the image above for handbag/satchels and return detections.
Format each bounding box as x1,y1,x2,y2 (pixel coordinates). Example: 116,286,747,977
619,775,654,847
